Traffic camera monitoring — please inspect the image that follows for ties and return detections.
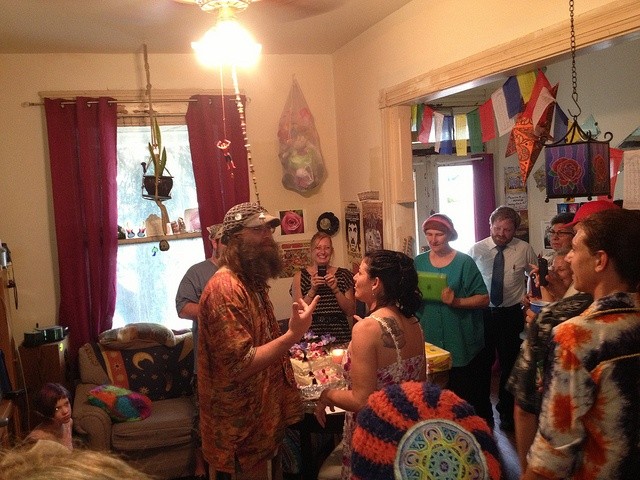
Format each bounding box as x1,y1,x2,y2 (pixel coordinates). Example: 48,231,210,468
260,290,295,386
491,246,506,307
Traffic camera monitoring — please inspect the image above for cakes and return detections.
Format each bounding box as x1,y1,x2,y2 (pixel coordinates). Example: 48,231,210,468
286,346,350,399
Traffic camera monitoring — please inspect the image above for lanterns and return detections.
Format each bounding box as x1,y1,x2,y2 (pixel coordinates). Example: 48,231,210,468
535,115,613,203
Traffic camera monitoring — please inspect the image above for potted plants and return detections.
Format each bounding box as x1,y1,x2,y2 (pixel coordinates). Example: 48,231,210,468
141,116,174,197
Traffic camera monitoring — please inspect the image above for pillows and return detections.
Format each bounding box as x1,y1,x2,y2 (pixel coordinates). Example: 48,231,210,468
93,332,194,402
78,343,111,386
96,323,176,350
87,384,153,422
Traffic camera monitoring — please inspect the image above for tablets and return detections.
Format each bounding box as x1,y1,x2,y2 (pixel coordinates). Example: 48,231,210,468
417,271,447,302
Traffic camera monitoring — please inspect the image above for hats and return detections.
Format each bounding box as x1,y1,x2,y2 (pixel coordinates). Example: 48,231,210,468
559,200,620,228
221,202,281,245
422,213,458,241
351,380,502,480
206,224,223,240
316,212,339,236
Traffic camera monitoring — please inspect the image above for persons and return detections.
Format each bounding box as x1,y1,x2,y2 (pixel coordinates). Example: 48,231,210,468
289,231,355,348
522,207,638,479
551,249,572,288
541,212,576,270
345,203,361,258
504,293,594,474
313,248,427,480
216,138,238,170
525,200,619,328
175,222,222,480
414,212,490,410
195,200,321,480
466,205,538,428
26,383,75,451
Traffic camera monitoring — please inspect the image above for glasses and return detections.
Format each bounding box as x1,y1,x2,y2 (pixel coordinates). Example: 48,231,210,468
234,226,275,235
314,246,331,250
548,229,572,237
423,233,445,238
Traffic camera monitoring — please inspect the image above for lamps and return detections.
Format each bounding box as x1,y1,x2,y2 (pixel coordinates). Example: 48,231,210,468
189,1,263,74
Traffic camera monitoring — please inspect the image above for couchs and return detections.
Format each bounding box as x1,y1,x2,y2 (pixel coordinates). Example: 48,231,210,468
71,323,197,477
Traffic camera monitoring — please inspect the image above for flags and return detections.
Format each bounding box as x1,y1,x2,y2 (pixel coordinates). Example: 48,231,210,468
388,69,551,159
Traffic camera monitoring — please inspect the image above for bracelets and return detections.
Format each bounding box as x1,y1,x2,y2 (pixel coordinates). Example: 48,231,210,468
306,293,314,299
332,286,340,294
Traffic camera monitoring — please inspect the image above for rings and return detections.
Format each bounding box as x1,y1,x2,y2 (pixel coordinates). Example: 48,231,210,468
313,411,319,416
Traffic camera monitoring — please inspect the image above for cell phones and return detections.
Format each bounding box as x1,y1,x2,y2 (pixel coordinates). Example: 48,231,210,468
318,264,327,280
531,274,542,298
538,258,548,287
